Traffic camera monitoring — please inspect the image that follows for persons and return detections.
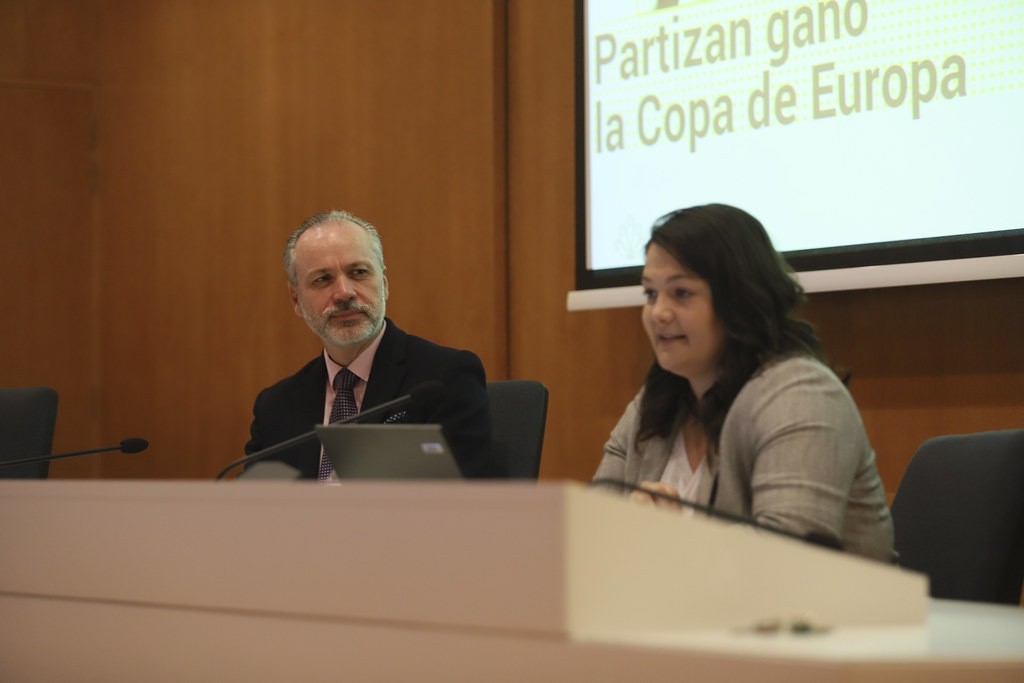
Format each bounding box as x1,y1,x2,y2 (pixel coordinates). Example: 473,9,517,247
242,209,492,480
588,203,895,566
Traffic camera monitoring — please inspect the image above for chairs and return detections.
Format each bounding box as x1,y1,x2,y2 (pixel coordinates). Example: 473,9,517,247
0,386,59,481
889,429,1024,609
486,379,550,481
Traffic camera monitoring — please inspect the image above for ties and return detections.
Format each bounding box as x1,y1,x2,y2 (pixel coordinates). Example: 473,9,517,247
318,367,361,482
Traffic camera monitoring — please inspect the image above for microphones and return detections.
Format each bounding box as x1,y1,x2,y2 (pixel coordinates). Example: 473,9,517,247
0,438,150,469
216,380,442,481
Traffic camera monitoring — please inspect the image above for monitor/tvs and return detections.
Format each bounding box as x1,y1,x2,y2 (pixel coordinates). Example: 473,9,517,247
571,0,1024,291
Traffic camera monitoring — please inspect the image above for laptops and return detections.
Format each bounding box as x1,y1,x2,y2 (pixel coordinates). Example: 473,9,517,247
315,423,462,482
